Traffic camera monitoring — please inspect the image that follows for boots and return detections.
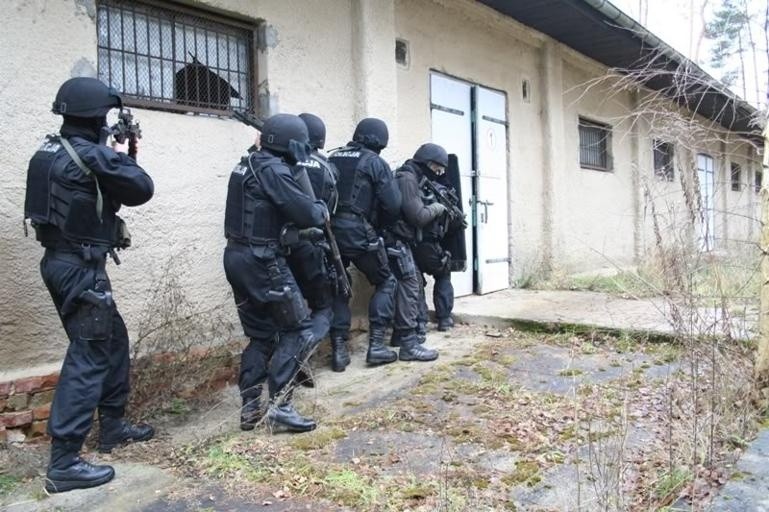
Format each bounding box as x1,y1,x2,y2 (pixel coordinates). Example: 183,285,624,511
95,412,155,451
43,434,116,493
265,391,318,433
364,327,398,368
329,334,352,373
391,321,440,362
237,393,266,432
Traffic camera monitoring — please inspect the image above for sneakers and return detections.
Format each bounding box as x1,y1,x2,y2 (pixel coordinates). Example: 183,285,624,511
436,316,456,331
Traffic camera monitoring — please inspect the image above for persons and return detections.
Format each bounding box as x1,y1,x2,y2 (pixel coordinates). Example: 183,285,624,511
376,141,450,363
326,116,405,374
413,178,455,332
20,75,156,494
222,112,334,435
282,111,341,388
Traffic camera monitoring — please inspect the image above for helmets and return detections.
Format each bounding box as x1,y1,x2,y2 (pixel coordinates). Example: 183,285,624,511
50,77,119,120
257,112,309,156
350,117,391,150
413,142,451,168
295,113,326,152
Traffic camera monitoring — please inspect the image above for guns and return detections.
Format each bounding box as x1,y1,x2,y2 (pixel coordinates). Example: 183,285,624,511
294,166,356,300
417,175,469,230
